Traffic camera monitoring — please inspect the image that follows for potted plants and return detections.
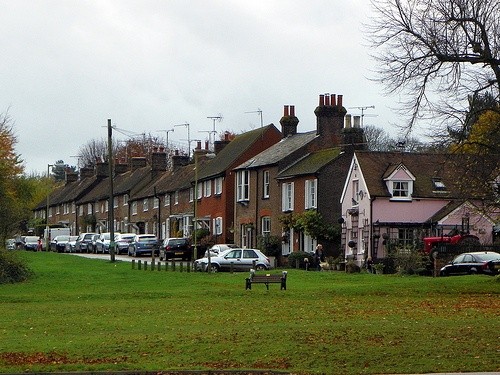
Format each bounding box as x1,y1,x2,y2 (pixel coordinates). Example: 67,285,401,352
374,263,385,275
319,255,360,273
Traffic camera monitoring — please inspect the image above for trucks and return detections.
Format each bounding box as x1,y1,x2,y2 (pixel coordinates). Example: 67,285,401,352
40,227,71,251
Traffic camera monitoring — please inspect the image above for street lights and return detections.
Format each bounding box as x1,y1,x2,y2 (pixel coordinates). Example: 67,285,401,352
46,163,54,228
193,153,217,262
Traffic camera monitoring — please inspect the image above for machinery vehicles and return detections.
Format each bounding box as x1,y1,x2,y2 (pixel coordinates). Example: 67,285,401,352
423,214,480,256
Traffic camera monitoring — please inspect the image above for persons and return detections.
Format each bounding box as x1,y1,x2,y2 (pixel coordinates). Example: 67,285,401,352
316,244,324,271
37,239,42,251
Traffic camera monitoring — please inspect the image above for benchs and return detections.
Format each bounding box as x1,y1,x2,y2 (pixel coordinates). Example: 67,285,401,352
245,271,287,291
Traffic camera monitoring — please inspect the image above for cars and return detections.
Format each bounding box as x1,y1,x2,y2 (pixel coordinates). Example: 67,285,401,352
50,235,69,253
205,243,243,258
6,236,27,250
86,234,101,253
109,234,137,255
23,236,40,252
160,237,192,262
75,233,95,253
127,234,160,257
438,251,500,277
94,232,121,254
194,249,270,273
65,235,78,253
